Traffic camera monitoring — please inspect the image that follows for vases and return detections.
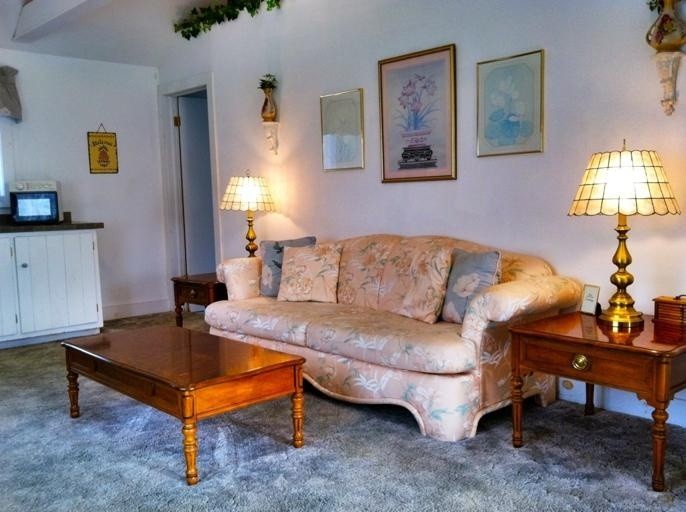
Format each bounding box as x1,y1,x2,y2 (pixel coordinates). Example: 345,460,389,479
643,0,685,53
400,127,434,149
261,88,277,123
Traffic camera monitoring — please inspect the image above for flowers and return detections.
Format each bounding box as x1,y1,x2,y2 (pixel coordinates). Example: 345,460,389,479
396,71,442,129
255,71,279,89
490,75,533,143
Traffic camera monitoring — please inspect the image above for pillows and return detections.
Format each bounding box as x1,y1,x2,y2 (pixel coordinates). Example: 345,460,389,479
440,246,504,324
332,234,404,311
276,241,347,304
377,238,454,325
258,235,318,298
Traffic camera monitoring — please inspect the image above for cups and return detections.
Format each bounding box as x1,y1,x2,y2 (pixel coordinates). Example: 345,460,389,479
63,211,71,225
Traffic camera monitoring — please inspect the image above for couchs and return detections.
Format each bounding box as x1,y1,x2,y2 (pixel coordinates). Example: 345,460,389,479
202,232,582,445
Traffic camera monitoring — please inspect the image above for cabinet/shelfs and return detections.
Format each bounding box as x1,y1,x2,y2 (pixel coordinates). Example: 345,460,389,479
0,220,106,349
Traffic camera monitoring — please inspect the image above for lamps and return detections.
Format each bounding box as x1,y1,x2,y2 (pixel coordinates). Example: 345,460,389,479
217,168,278,257
565,136,682,330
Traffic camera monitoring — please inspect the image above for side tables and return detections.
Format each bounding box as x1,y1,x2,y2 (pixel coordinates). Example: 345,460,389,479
170,271,228,328
507,309,686,493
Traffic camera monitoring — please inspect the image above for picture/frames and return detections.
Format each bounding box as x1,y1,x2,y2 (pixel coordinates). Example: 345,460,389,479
475,48,545,157
578,282,603,317
318,87,365,172
377,43,459,184
